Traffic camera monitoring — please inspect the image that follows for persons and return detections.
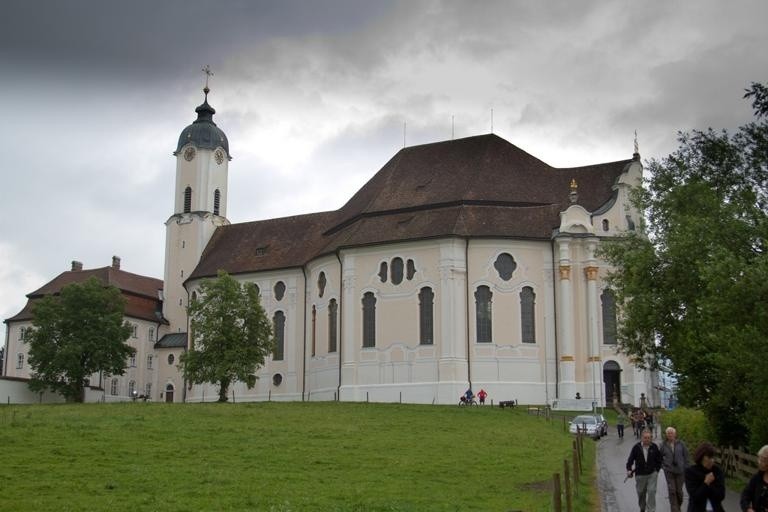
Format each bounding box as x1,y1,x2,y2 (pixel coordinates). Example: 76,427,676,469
463,387,473,400
626,427,662,511
739,444,768,511
477,388,488,405
659,426,690,511
683,441,727,511
617,407,658,441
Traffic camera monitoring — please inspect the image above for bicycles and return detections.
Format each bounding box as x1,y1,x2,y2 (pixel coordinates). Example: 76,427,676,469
458,398,477,409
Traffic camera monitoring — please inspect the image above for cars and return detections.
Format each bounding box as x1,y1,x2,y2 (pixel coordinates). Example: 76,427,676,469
566,414,602,440
583,412,608,437
